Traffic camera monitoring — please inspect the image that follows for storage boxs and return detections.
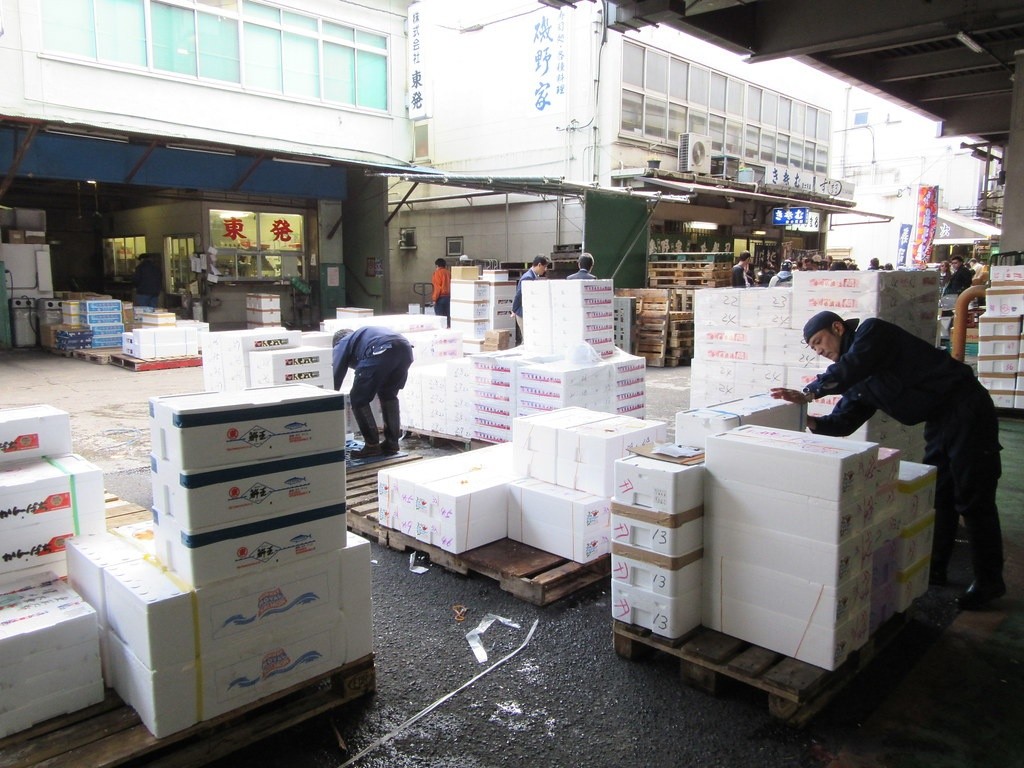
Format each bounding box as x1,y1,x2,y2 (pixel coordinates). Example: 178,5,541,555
0,266,939,740
948,327,978,344
978,265,1024,409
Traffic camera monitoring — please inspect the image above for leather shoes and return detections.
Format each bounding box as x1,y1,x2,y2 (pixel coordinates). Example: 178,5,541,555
928,572,946,586
955,575,1007,606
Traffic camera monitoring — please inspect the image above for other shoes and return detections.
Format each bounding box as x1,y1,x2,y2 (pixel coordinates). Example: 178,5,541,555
351,444,384,458
381,441,400,454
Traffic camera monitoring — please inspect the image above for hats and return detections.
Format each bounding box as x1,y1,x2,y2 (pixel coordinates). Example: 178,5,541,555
812,254,822,262
803,311,845,345
781,259,793,272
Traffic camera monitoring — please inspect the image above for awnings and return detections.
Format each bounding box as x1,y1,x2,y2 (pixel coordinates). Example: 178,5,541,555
635,176,894,226
0,109,691,280
933,211,1001,244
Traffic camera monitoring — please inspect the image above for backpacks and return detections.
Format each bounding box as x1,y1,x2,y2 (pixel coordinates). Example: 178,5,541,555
774,274,792,287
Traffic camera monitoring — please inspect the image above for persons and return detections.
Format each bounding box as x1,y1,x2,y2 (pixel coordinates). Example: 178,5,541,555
135,253,162,307
937,256,985,317
731,250,754,287
868,258,893,270
771,311,1007,611
432,258,450,327
238,255,252,276
567,253,597,280
511,255,550,332
761,255,859,287
333,326,413,459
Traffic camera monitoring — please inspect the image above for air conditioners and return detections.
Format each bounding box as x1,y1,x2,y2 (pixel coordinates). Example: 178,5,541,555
677,133,712,174
